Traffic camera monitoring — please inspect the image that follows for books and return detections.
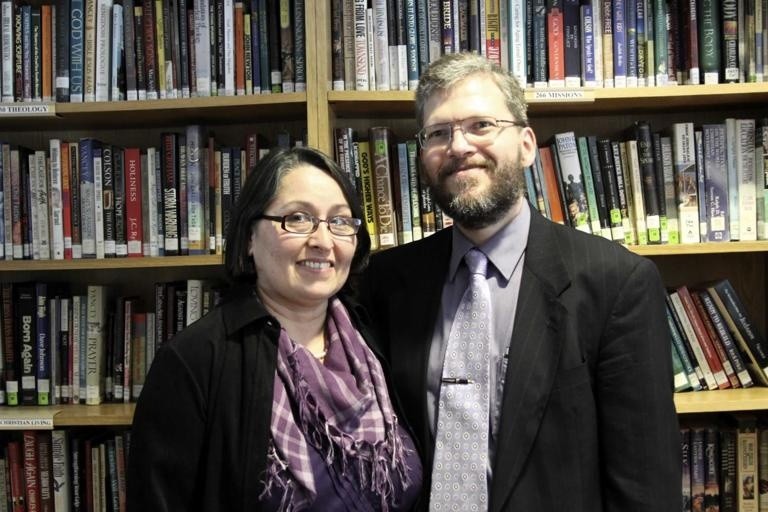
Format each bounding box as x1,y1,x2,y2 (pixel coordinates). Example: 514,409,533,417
523,119,768,245
665,276,768,391
0,0,305,103
681,418,768,512
330,0,768,90
2,280,224,406
334,127,454,250
1,124,308,260
1,426,130,512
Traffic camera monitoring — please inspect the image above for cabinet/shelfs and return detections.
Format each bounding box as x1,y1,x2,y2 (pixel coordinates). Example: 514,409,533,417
0,2,315,430
314,0,768,410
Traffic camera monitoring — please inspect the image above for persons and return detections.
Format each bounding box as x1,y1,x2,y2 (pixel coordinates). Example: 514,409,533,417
125,149,425,512
336,53,682,512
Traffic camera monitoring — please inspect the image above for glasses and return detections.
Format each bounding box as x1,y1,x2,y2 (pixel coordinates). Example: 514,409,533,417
253,214,363,237
414,116,525,152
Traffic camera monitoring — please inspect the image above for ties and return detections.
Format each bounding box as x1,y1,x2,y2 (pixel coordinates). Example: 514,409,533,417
428,249,490,512
